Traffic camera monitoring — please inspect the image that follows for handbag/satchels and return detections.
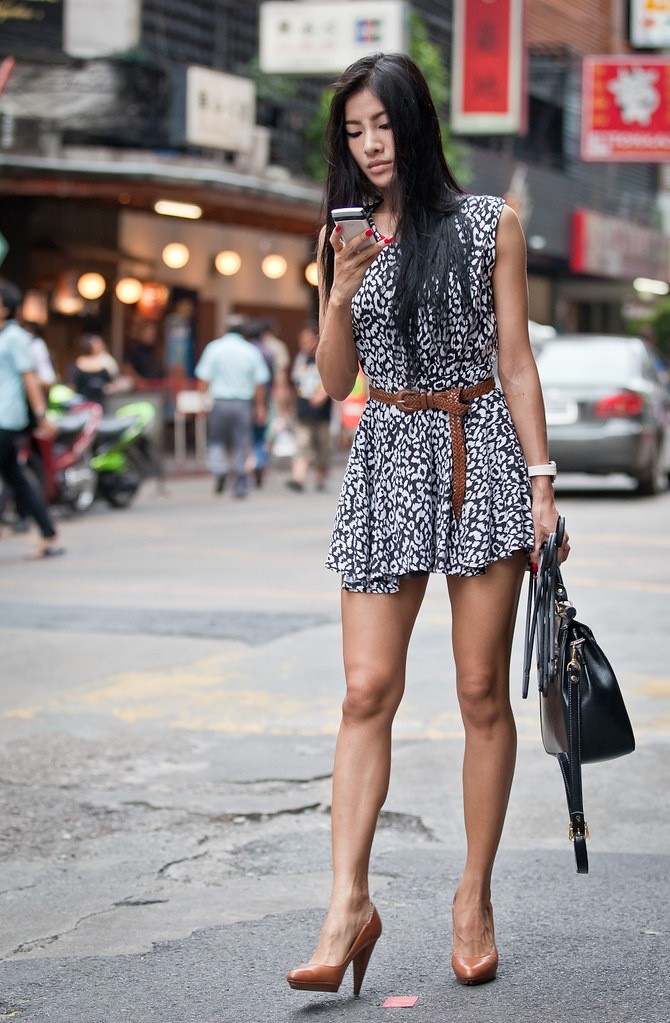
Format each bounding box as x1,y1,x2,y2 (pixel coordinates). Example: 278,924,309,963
522,516,636,875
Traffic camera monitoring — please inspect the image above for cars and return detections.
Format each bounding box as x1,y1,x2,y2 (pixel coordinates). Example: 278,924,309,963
530,334,669,493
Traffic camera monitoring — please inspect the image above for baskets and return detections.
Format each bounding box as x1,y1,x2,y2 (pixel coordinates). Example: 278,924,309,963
369,377,496,519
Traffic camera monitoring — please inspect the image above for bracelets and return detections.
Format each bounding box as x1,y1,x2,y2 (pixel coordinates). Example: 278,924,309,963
33,410,47,419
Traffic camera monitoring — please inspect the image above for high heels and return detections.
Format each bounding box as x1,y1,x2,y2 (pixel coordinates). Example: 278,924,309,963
288,903,382,997
451,890,498,985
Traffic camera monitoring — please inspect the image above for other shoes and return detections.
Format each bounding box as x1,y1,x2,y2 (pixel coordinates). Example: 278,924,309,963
28,547,65,559
214,461,324,498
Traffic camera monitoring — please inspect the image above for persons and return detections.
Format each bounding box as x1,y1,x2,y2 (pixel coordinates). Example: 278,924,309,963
272,55,574,999
256,319,290,406
64,335,121,406
286,326,331,493
0,278,66,558
121,318,167,383
194,313,271,501
23,318,57,389
242,323,274,489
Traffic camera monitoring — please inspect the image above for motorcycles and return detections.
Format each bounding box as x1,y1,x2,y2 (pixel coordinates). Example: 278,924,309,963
1,381,156,532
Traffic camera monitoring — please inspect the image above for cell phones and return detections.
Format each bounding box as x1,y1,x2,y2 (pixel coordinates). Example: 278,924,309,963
331,206,378,254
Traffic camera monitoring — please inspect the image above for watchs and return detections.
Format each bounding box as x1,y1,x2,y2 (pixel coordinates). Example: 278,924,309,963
528,460,558,484
